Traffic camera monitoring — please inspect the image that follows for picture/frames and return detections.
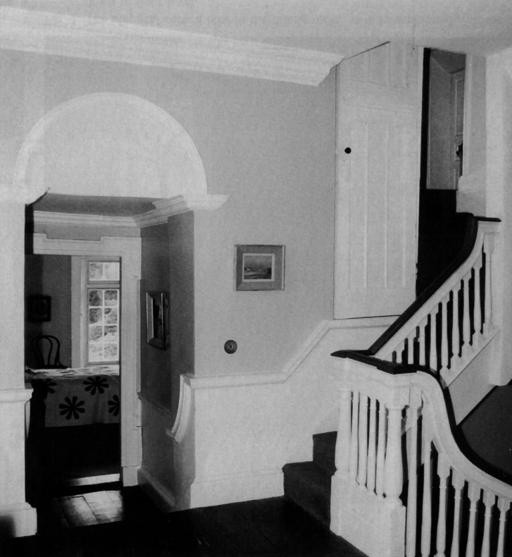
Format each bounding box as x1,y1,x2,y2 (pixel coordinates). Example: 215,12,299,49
235,244,285,291
146,290,169,350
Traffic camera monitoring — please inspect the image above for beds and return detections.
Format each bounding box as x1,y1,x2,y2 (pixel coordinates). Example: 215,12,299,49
25,364,121,427
